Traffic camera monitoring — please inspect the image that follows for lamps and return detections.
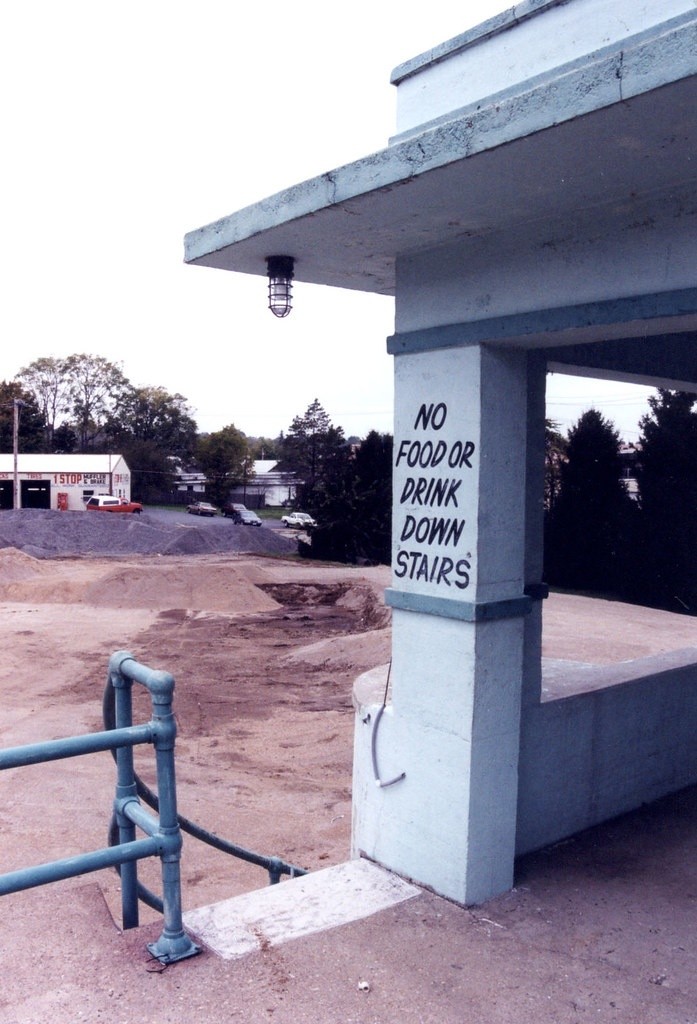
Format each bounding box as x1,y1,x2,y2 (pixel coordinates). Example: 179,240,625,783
267,255,296,318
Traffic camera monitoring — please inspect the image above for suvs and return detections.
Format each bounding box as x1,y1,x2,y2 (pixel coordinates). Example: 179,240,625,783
85,495,144,516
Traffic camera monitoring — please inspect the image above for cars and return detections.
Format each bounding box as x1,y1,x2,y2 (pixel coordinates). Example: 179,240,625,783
221,503,248,517
281,512,317,531
185,501,217,517
233,511,262,527
